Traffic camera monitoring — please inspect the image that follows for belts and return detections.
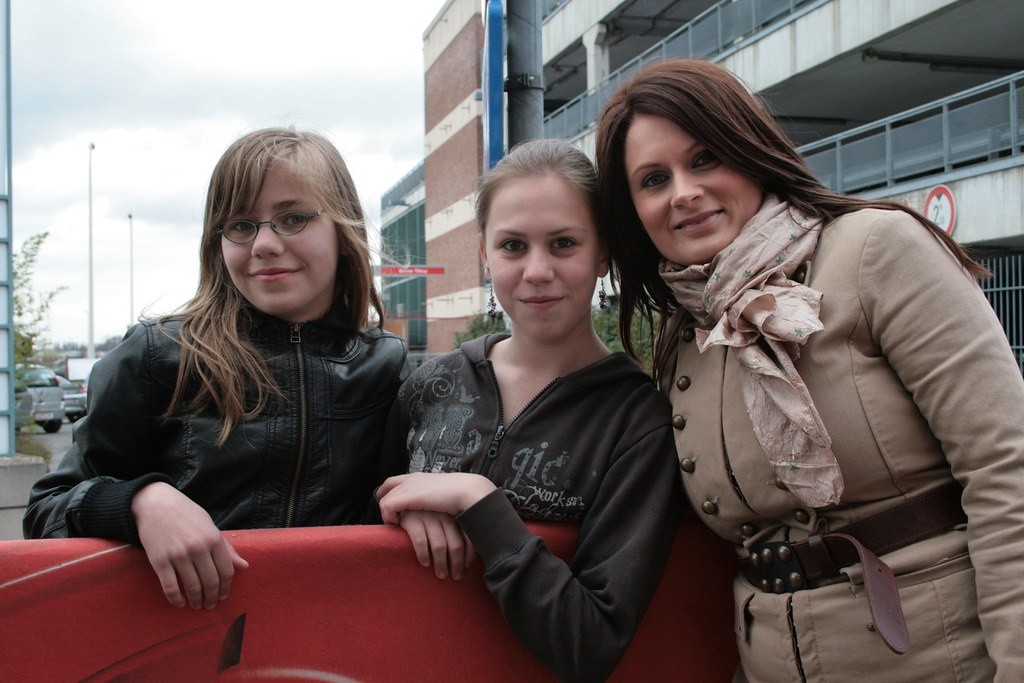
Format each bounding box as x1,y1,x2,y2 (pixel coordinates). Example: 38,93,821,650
735,481,964,655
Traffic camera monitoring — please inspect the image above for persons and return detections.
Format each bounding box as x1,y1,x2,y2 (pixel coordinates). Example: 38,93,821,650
23,124,413,613
597,59,1023,682
376,137,684,683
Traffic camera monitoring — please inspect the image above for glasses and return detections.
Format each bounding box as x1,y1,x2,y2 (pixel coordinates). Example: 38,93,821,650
216,206,325,244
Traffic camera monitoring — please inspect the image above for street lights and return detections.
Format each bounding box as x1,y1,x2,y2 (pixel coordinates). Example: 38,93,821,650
129,214,133,327
88,144,95,359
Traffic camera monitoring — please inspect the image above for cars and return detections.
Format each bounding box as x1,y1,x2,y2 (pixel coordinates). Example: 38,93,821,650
41,374,88,423
14,363,65,433
